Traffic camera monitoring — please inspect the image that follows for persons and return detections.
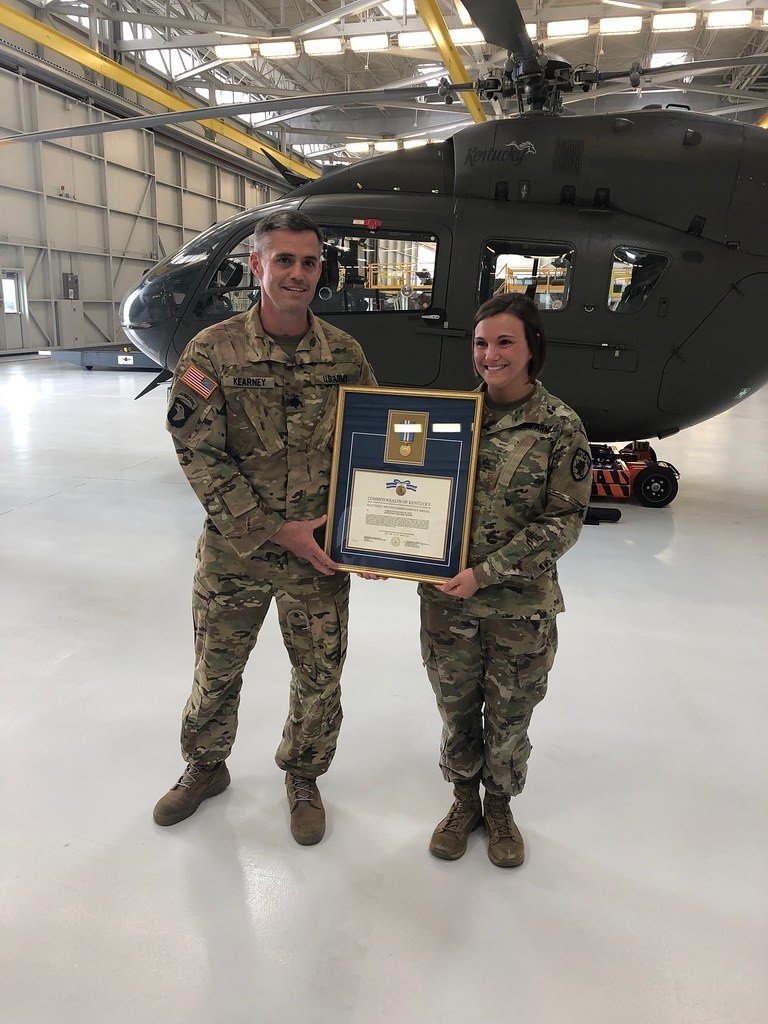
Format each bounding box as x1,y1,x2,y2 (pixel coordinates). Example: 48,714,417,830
152,208,384,847
415,289,592,868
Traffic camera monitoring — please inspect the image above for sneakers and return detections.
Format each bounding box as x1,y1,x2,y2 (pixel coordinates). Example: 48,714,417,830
153,761,231,826
482,789,525,868
429,783,481,860
285,771,326,847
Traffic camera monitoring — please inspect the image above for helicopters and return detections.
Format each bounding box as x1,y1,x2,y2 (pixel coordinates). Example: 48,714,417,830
0,1,768,505
